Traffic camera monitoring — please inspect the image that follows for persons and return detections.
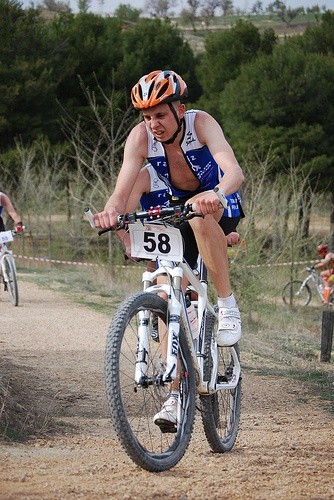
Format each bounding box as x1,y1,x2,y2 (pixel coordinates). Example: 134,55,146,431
0,192,23,252
306,244,334,305
92,71,246,425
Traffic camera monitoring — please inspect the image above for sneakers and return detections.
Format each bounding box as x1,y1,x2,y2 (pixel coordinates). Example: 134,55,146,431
153,396,178,426
151,315,159,343
217,307,241,346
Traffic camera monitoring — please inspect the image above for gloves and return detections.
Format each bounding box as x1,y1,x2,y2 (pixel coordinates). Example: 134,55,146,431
14,222,23,232
306,265,315,271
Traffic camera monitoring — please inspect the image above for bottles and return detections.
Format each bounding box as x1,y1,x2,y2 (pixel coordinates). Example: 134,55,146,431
181,299,200,342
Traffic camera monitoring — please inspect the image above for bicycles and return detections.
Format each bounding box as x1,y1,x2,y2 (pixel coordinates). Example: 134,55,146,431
0,226,29,307
280,265,334,311
82,186,242,473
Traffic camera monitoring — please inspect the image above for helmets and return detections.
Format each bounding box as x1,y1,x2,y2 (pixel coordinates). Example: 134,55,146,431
131,70,188,110
317,245,328,252
